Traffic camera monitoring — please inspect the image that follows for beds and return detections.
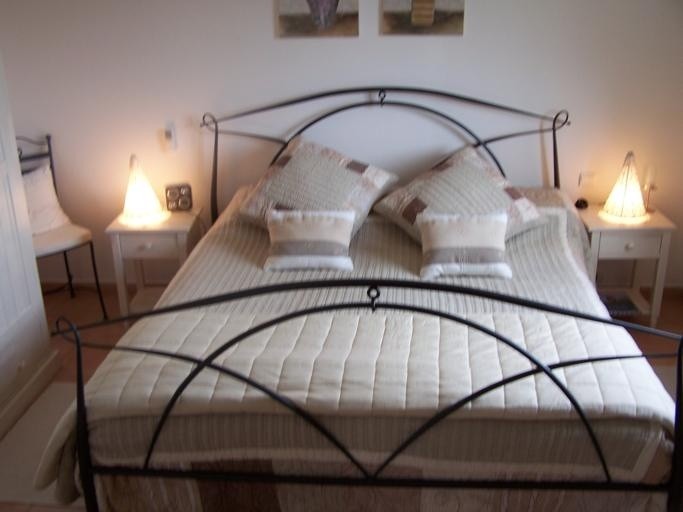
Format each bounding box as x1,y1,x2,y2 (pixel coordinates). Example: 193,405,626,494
33,86,680,512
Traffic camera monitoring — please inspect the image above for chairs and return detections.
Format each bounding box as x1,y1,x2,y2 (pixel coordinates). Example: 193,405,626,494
13,133,110,321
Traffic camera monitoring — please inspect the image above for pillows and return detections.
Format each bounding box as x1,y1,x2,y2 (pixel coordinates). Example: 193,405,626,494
262,208,359,273
416,212,514,281
372,144,547,249
239,138,399,240
20,164,71,236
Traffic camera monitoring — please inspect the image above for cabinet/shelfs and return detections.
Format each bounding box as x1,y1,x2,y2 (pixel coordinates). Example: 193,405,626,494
0,55,63,443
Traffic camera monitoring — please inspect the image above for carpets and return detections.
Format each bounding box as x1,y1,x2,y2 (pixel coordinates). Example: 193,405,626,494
0,358,678,509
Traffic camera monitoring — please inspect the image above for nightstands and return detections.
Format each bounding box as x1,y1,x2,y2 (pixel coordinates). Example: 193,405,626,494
103,207,205,327
580,205,676,327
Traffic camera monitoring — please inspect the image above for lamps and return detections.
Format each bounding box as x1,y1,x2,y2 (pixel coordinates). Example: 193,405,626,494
117,155,167,228
602,149,650,225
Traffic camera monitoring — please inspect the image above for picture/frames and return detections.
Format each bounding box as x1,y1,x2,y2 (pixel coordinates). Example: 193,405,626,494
270,0,359,40
378,0,464,37
